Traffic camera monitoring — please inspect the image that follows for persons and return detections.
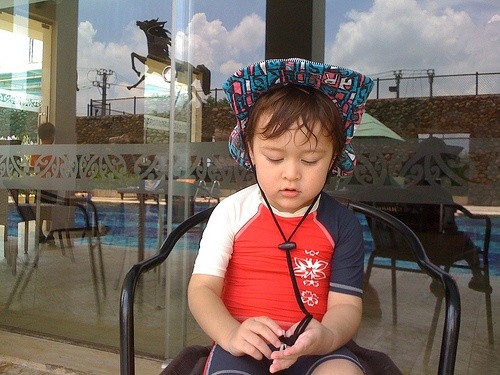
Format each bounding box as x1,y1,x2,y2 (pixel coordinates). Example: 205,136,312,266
188,58,374,375
30,121,66,242
404,137,492,299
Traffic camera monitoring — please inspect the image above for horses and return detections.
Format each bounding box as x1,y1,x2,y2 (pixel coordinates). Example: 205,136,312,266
126,17,212,107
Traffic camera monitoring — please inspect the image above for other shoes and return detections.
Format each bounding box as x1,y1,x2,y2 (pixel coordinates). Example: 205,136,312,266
39,234,55,242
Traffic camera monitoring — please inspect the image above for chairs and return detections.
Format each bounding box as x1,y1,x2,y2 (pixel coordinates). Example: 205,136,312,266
3,179,107,316
359,200,493,365
118,193,461,375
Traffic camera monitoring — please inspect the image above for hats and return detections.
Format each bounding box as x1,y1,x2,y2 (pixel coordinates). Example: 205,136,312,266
222,57,376,177
